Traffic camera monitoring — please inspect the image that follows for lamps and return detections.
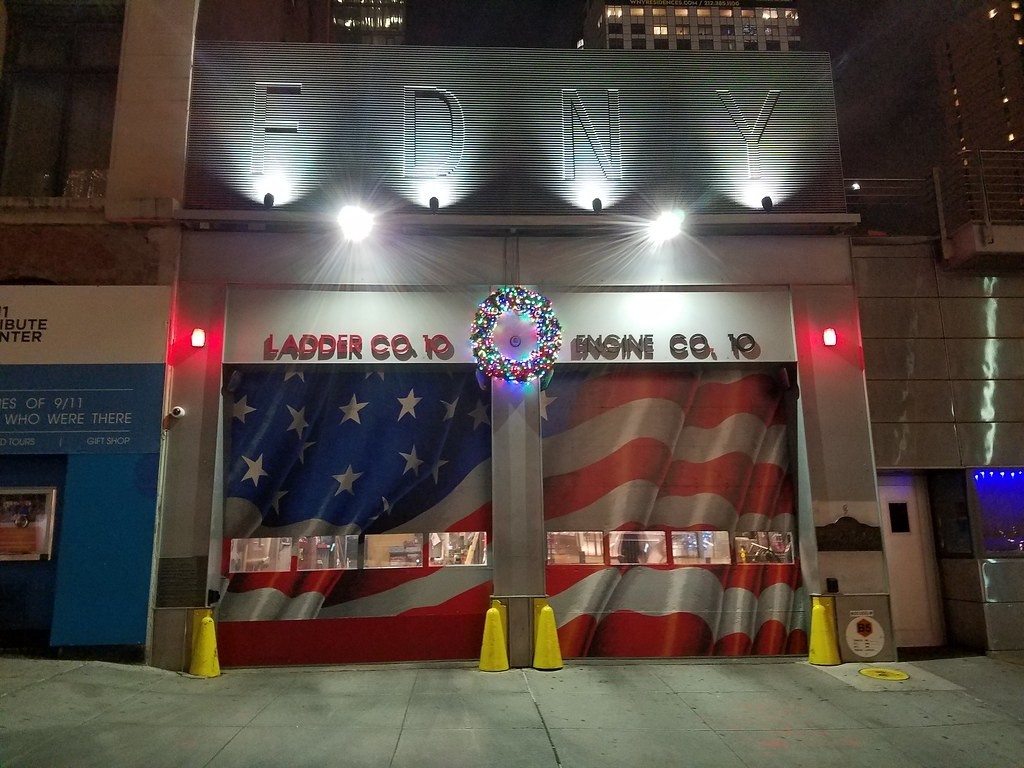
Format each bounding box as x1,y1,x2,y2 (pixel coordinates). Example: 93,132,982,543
264,193,275,210
430,197,439,215
761,196,773,214
593,198,602,215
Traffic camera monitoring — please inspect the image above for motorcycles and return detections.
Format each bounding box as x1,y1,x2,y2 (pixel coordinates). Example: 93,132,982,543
12,513,29,528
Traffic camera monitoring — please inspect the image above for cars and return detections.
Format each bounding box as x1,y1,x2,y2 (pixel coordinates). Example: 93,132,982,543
663,539,715,557
